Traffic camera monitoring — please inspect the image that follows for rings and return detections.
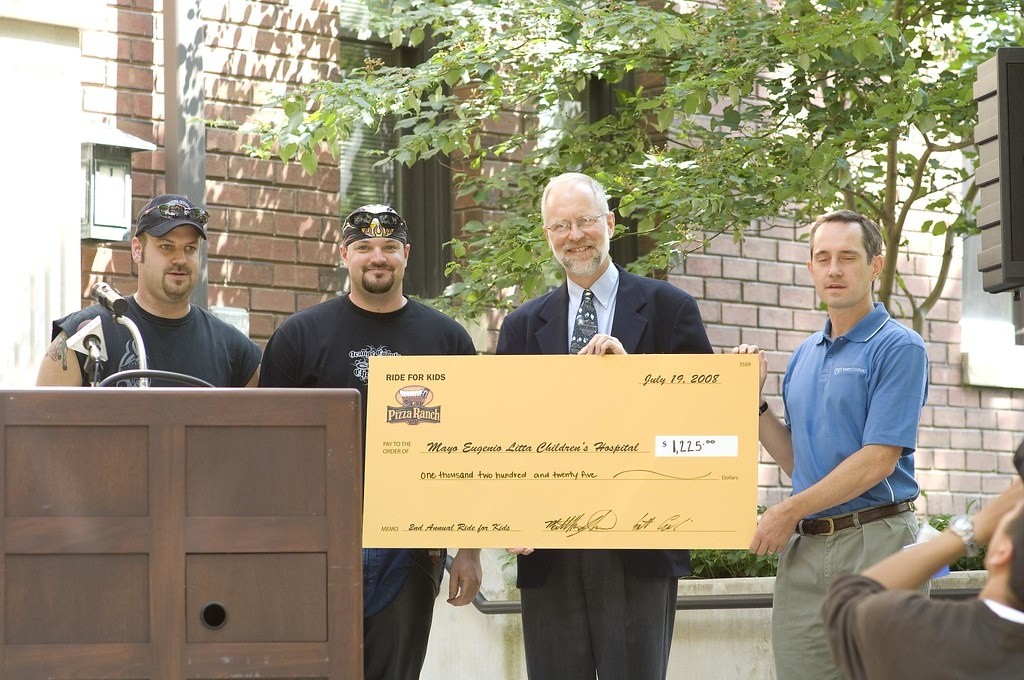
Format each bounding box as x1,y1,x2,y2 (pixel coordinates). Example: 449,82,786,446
767,549,774,555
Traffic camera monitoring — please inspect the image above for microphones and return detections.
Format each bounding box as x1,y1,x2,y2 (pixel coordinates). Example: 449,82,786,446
66,316,108,362
90,281,129,316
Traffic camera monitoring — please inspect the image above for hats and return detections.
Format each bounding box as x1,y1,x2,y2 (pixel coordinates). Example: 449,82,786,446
134,193,211,240
341,204,409,245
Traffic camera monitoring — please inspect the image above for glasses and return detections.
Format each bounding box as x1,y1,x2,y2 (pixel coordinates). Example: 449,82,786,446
342,211,405,229
137,202,210,225
545,214,605,232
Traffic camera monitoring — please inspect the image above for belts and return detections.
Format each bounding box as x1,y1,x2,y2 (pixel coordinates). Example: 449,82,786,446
794,500,914,536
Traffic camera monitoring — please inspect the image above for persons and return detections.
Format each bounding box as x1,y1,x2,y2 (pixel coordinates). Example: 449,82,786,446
729,211,930,680
494,174,714,680
821,444,1024,680
255,206,482,680
36,197,264,387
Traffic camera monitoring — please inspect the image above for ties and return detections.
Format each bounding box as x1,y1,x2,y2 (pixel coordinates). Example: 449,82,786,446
567,288,604,356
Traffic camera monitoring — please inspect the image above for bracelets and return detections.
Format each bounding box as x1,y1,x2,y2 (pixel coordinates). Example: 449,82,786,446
759,401,768,414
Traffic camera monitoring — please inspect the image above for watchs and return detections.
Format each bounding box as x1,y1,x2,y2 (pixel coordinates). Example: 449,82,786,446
948,516,977,558
